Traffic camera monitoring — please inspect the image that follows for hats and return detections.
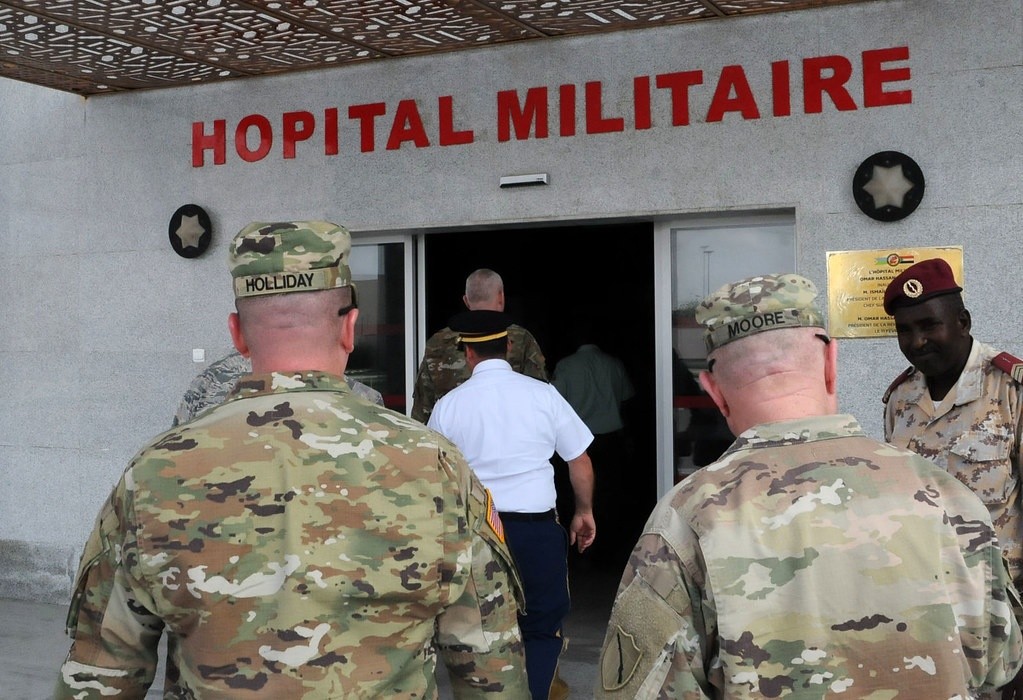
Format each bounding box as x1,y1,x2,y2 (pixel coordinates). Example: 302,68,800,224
694,275,827,354
448,310,509,352
230,218,353,298
883,258,963,316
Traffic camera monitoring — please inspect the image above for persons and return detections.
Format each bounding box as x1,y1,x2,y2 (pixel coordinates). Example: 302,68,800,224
163,353,386,700
883,258,1023,634
411,269,548,426
594,274,1023,700
51,222,532,700
550,334,636,564
426,310,596,700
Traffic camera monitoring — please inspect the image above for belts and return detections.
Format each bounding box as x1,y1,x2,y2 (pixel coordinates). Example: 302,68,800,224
499,509,556,522
